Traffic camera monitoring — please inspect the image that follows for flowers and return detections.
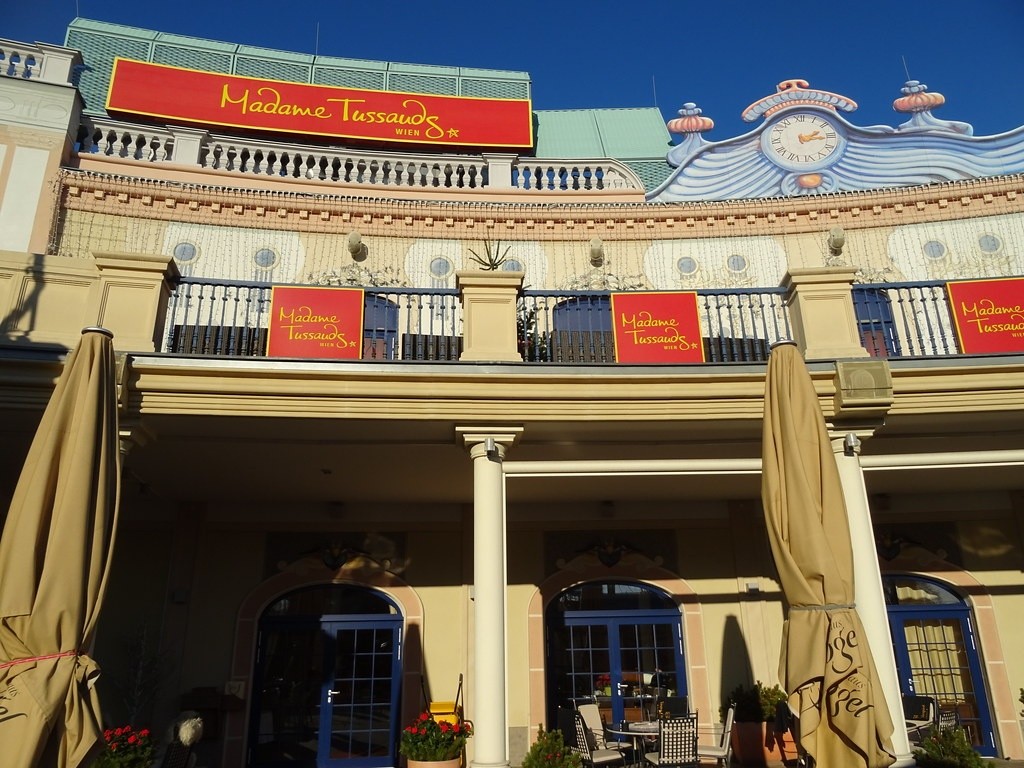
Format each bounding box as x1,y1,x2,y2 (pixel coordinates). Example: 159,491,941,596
96,726,153,767
399,714,474,762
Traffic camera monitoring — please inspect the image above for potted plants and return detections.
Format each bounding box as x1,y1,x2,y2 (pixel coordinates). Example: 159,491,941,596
718,679,800,761
408,757,461,768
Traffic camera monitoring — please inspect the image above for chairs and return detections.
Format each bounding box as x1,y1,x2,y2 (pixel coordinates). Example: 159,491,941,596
645,708,701,768
917,706,958,743
577,704,632,768
606,724,659,768
553,709,626,768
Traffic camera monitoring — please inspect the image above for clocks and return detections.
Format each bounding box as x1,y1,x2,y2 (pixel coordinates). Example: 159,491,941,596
760,110,848,171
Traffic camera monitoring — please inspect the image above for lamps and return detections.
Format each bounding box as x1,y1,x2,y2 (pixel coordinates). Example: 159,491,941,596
589,239,603,260
348,230,362,256
829,226,845,254
